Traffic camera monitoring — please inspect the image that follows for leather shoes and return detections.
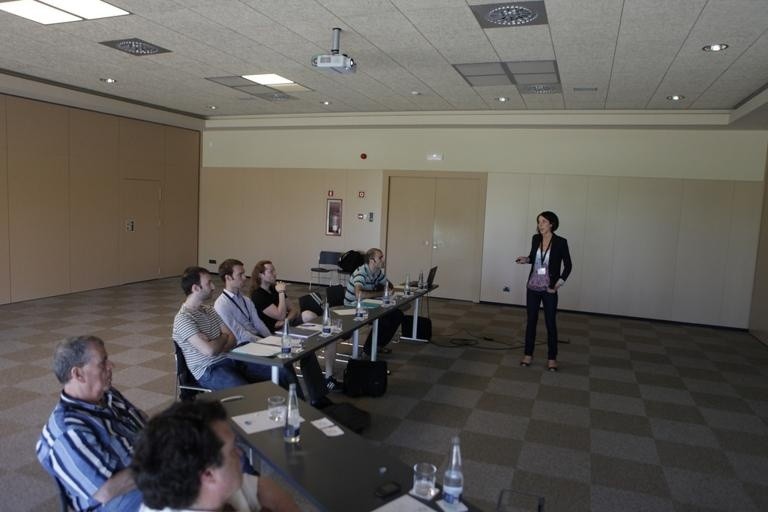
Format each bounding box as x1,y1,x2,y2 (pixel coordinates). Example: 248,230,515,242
310,397,338,410
361,351,379,359
380,347,393,355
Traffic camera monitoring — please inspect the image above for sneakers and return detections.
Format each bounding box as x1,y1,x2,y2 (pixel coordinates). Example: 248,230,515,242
324,375,344,391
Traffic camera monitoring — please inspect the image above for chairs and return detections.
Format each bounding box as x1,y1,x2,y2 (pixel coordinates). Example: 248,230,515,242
309,250,344,290
298,292,349,364
172,340,212,402
52,475,77,512
326,284,364,358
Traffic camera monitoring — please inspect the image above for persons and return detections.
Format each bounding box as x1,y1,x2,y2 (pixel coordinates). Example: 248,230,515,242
516,211,572,372
171,267,249,391
344,249,404,355
129,402,241,511
302,301,344,391
248,261,335,411
35,337,300,512
214,258,305,401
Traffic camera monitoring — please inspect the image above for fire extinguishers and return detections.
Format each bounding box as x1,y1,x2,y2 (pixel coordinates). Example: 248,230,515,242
331,209,340,232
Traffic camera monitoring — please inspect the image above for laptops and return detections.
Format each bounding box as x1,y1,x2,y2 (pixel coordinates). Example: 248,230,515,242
401,266,438,288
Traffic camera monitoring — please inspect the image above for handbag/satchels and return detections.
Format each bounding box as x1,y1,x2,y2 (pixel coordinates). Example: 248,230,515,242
400,315,433,343
342,357,387,397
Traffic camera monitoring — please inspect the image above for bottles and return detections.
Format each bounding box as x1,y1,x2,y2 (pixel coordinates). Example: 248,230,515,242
417,270,423,292
355,291,363,321
280,319,291,358
322,303,331,337
403,273,410,297
441,436,463,511
382,281,390,307
283,385,300,442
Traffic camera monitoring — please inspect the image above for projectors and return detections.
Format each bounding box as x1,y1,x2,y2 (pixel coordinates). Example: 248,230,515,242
310,52,356,73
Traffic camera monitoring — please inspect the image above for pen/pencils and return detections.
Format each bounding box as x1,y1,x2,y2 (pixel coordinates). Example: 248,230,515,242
221,395,244,404
302,325,316,327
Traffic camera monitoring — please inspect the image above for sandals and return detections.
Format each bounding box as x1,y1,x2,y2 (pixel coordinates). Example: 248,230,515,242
546,358,559,372
520,354,534,368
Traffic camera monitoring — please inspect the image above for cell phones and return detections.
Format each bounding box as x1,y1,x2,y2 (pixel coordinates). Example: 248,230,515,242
375,480,400,497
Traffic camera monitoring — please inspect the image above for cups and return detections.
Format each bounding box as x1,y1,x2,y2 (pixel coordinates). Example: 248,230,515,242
290,339,302,353
267,396,285,418
412,462,437,497
332,319,342,333
362,307,368,319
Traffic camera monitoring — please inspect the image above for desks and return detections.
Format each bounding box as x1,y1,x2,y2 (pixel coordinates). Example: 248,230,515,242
195,380,483,512
226,280,440,385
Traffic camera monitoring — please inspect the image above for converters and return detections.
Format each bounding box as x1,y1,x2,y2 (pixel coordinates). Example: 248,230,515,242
483,337,495,342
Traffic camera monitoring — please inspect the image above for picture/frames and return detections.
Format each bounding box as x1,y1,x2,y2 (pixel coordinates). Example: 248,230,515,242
324,198,343,236
